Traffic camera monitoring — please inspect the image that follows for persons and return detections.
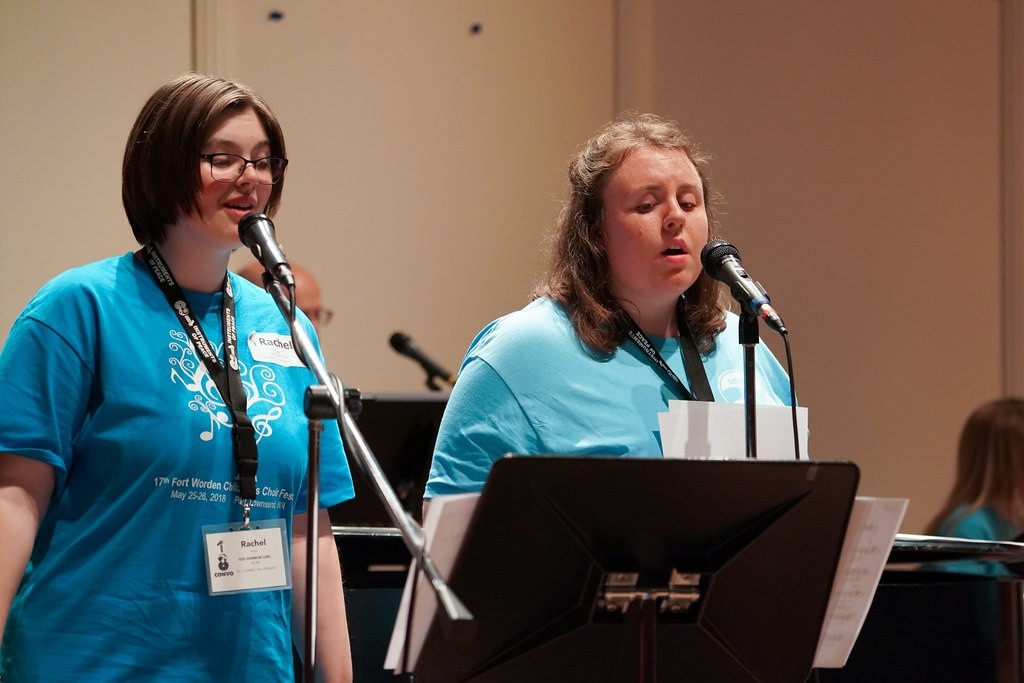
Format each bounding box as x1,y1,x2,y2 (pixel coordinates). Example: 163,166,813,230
917,398,1024,578
1,74,357,683
420,112,797,527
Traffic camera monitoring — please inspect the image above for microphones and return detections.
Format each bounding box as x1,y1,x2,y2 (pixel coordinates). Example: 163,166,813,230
238,211,296,289
700,238,788,335
389,331,457,386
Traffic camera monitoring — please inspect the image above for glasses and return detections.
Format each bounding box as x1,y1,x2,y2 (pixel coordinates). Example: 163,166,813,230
199,152,289,185
